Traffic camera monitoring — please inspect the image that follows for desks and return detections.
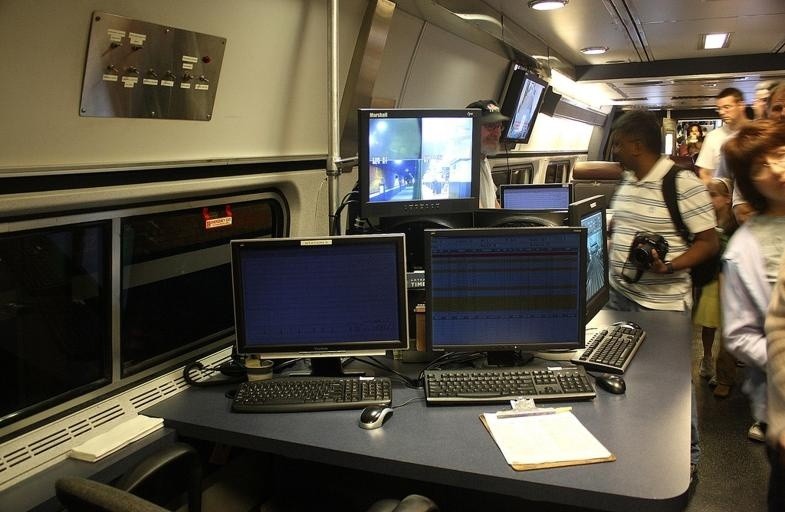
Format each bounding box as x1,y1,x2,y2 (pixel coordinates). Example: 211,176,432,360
136,307,696,512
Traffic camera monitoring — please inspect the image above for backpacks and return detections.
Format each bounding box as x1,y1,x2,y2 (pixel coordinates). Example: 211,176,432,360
661,164,721,288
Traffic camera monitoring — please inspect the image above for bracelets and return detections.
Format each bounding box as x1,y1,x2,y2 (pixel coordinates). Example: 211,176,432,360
667,263,674,274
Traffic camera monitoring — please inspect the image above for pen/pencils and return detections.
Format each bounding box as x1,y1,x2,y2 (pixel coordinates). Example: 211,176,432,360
497,407,572,419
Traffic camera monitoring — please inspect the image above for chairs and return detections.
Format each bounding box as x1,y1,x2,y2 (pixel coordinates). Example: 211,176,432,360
54,442,441,512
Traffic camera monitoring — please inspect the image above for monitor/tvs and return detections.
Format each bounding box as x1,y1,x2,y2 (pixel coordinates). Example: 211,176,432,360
356,108,480,216
499,70,548,144
423,227,587,365
499,183,572,210
569,193,610,325
228,233,409,377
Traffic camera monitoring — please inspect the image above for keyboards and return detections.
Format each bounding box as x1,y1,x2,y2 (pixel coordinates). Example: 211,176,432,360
406,271,426,289
572,328,647,374
423,365,596,406
232,376,392,413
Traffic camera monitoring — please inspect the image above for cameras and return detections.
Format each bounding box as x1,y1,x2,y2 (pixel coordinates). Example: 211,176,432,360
629,231,669,267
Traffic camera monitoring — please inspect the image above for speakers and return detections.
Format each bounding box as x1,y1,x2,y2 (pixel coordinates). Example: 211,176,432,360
380,212,473,273
475,209,567,229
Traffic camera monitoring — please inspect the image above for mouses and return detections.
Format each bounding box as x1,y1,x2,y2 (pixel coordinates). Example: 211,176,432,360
596,374,626,394
611,321,641,332
358,404,394,429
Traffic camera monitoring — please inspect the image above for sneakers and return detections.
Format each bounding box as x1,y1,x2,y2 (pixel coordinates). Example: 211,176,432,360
713,383,730,396
747,421,765,442
698,357,715,378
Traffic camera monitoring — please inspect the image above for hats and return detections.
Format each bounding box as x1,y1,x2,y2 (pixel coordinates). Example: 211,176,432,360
465,99,510,124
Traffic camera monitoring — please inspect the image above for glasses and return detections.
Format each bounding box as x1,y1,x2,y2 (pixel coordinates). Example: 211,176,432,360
486,125,505,132
714,105,737,112
749,151,785,182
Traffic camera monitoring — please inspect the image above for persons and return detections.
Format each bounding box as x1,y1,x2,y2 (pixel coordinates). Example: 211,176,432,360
608,109,720,316
465,100,509,210
676,78,785,512
